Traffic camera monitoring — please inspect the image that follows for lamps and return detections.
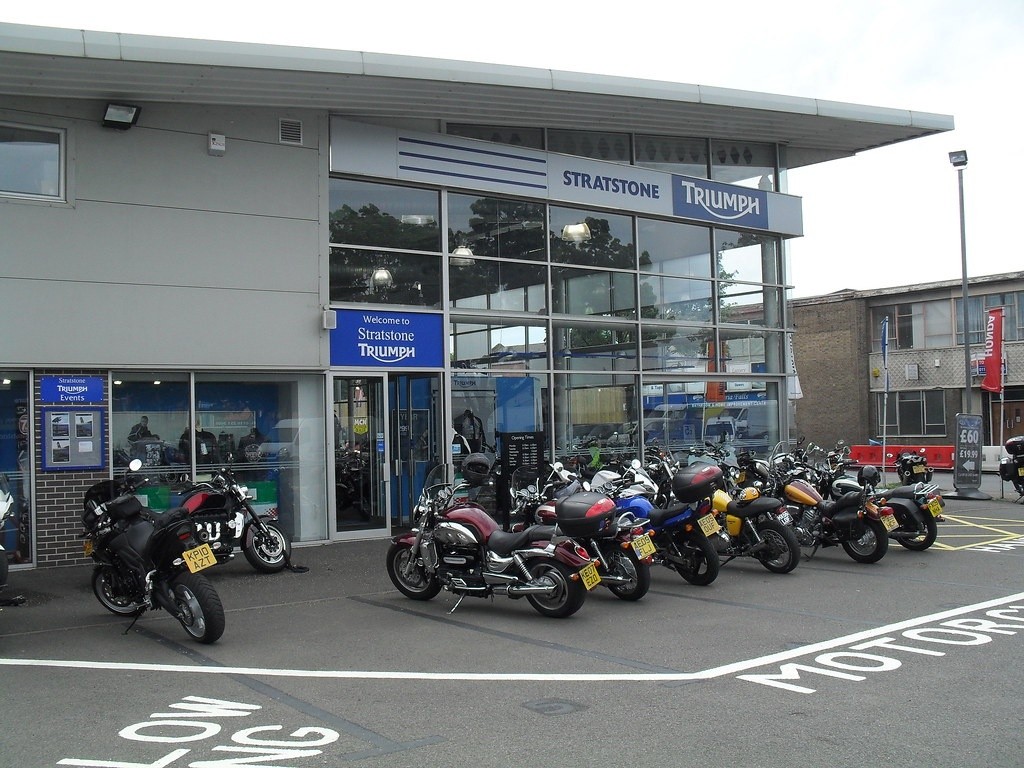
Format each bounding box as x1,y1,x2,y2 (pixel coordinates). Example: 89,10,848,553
562,222,591,241
370,267,393,285
450,246,476,265
101,101,139,130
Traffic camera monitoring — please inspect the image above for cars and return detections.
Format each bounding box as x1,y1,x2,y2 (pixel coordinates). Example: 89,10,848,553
550,397,772,443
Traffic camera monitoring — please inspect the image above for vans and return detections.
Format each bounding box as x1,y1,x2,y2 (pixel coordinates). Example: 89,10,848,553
255,417,325,467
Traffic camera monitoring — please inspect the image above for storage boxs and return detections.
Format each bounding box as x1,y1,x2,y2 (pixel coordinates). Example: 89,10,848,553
556,492,616,539
673,463,724,505
1004,435,1024,454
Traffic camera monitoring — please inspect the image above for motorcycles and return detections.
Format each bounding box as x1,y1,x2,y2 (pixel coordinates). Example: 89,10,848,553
385,462,602,620
999,435,1024,505
543,437,947,587
504,462,657,603
137,450,293,575
332,436,375,523
77,458,226,645
886,447,934,484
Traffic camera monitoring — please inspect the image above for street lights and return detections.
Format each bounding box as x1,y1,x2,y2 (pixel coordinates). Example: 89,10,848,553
949,148,973,414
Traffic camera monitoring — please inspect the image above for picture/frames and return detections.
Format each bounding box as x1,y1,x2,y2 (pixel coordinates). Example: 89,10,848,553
41,406,106,470
